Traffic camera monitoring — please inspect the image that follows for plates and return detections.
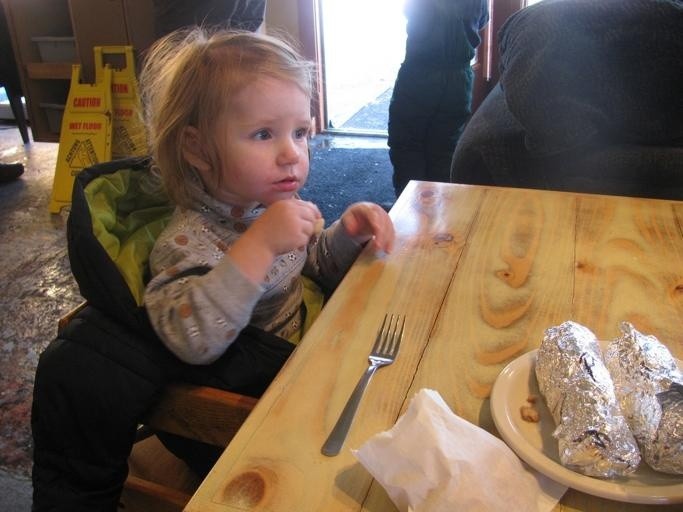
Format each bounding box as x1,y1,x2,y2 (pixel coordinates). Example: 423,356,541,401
489,340,682,504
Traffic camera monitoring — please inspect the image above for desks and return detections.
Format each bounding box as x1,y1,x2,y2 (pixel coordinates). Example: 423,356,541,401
183,179,682,511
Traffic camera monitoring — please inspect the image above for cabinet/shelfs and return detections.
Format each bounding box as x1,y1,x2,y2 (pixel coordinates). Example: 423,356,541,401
1,0,302,142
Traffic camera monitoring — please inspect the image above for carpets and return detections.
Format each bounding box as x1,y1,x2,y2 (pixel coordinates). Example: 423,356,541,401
299,149,396,225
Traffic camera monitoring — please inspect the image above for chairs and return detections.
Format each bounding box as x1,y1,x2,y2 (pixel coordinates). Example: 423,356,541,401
59,155,260,511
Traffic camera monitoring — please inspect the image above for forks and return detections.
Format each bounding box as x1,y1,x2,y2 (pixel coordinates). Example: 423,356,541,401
322,314,407,456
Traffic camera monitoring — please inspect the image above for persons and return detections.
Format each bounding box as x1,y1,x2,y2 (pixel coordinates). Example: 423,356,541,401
146,29,395,366
380,1,491,210
